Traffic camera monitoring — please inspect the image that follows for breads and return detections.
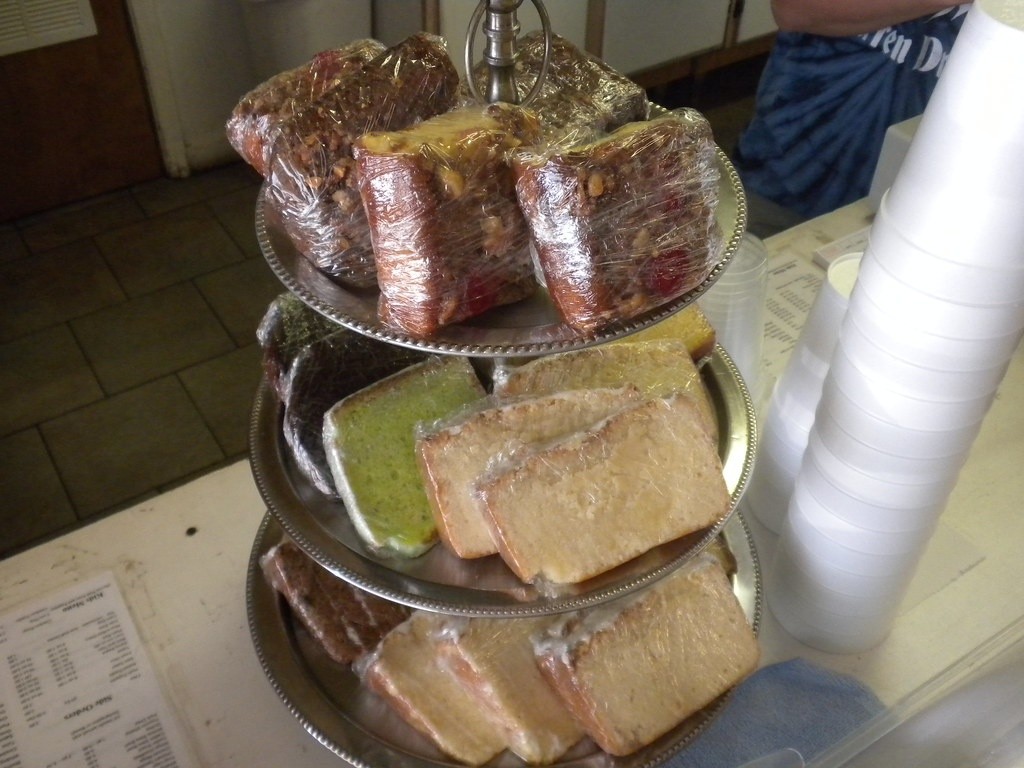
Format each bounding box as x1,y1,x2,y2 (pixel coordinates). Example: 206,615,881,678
263,540,759,768
256,290,733,586
227,32,716,336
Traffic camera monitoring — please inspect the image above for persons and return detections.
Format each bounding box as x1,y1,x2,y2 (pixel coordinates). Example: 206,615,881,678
729,1,979,221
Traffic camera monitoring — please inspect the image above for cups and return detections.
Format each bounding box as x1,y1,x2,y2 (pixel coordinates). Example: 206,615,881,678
763,0,1024,652
694,231,769,402
746,252,864,538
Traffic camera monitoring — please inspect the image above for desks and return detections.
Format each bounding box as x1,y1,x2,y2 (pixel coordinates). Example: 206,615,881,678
0,195,1024,768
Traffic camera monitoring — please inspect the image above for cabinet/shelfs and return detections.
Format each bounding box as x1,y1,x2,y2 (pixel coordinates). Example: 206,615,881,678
375,1,778,91
250,0,765,768
